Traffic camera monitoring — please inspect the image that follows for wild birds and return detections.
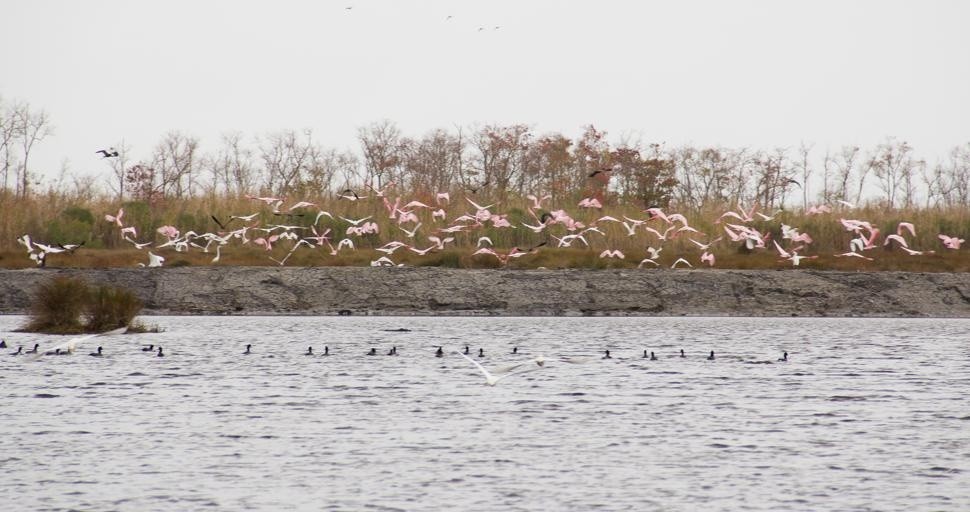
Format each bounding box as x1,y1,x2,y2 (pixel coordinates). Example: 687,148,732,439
588,167,612,177
15,233,86,267
626,203,966,268
456,356,543,386
96,150,119,158
102,181,627,268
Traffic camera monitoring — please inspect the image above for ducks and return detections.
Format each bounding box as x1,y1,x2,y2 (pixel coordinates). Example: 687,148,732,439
0,340,789,363
